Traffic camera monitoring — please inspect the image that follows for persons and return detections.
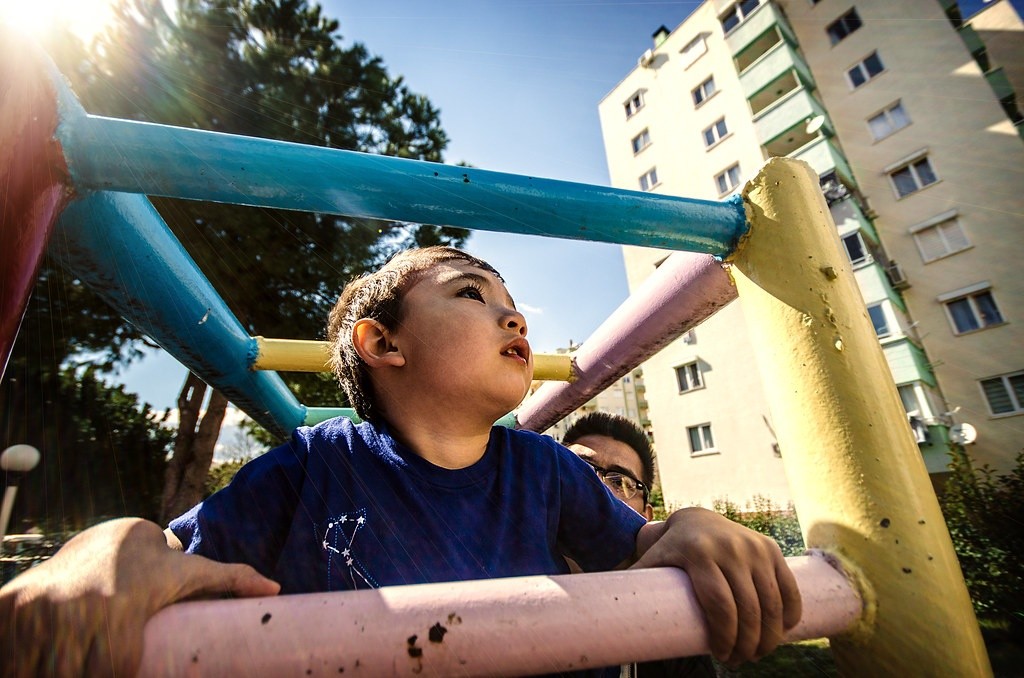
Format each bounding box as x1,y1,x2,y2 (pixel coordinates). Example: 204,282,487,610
0,244,804,678
551,410,717,678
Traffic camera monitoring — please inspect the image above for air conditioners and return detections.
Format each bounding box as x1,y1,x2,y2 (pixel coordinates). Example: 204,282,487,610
888,265,908,285
638,49,655,67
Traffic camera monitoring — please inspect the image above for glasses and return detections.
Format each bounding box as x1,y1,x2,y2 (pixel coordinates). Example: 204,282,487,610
586,461,649,506
